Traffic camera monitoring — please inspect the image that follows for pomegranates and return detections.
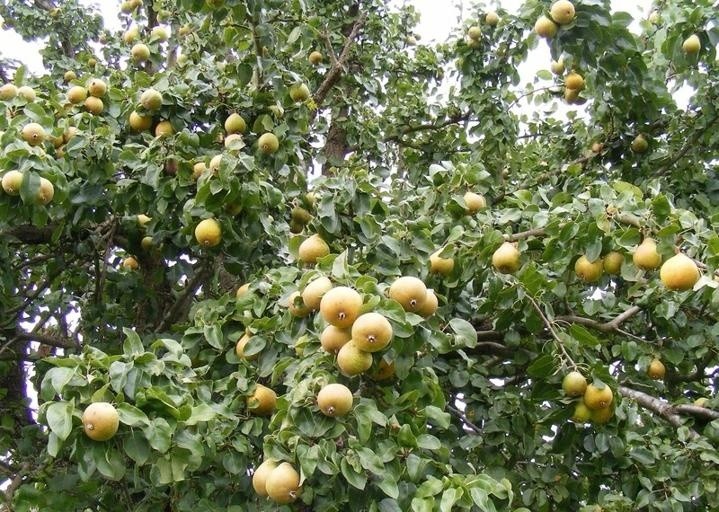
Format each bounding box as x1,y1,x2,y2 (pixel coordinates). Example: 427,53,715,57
0,0,719,505
